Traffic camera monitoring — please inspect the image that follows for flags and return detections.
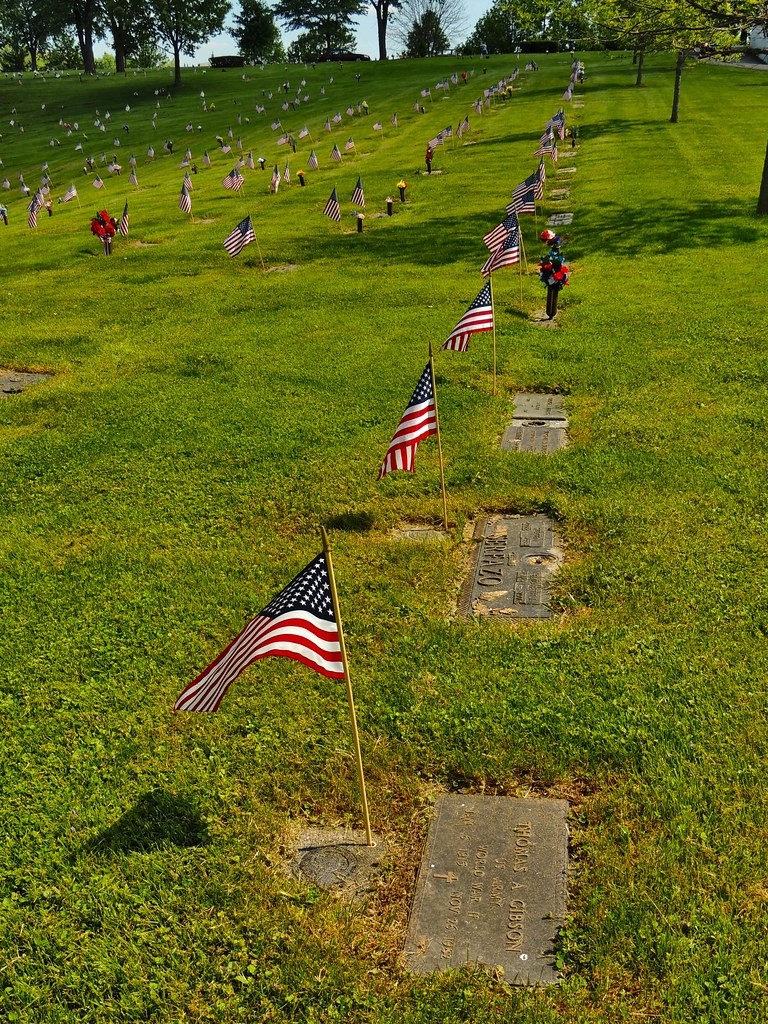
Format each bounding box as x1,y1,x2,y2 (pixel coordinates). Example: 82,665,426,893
436,281,493,354
119,203,128,238
171,556,349,732
479,219,521,279
0,60,586,223
482,212,518,255
376,361,437,480
27,203,36,228
222,216,256,258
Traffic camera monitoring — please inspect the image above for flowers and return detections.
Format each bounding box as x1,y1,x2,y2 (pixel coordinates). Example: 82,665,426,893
425,147,434,173
296,170,304,184
90,210,120,255
397,180,407,200
539,228,569,317
